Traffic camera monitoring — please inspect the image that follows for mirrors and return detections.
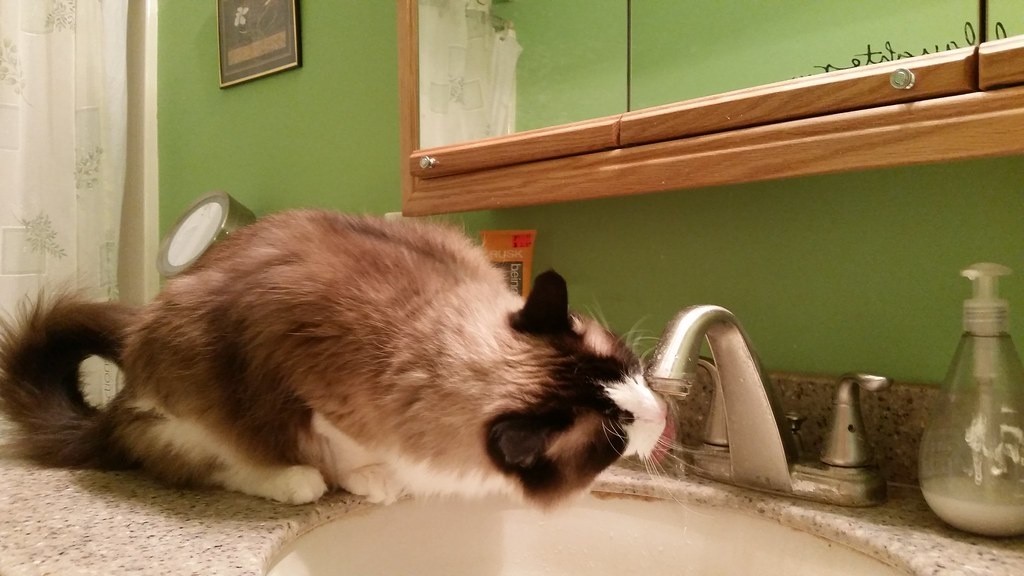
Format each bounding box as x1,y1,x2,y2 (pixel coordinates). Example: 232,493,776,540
397,1,1024,216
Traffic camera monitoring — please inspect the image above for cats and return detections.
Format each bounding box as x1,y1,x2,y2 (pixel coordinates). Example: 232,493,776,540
0,208,708,514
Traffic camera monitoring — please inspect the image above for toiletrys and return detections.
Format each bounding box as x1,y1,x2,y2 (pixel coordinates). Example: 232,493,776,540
912,259,1020,538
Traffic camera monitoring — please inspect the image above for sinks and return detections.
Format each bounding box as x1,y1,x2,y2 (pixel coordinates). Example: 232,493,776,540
255,469,935,576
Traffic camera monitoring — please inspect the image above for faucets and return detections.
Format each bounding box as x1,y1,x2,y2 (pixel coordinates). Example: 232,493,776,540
645,297,895,509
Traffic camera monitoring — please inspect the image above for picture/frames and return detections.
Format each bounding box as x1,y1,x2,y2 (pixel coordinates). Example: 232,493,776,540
215,0,302,88
